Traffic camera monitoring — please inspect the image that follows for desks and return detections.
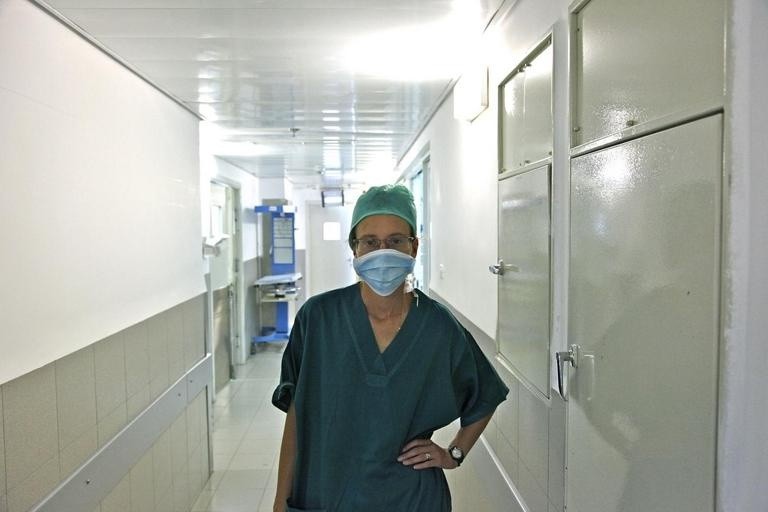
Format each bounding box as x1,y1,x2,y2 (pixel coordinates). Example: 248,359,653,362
249,270,303,355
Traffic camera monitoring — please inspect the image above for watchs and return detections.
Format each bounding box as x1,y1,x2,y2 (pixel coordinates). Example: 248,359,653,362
448,444,465,466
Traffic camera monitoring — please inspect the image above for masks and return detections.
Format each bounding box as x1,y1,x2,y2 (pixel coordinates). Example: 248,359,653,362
353,249,416,296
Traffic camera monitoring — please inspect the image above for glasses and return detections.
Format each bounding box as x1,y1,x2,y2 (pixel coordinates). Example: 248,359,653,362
352,236,416,251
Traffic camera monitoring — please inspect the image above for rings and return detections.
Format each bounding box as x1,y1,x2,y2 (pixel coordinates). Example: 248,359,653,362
425,452,431,460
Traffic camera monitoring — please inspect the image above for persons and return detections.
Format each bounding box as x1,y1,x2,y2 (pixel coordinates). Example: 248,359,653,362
270,183,510,512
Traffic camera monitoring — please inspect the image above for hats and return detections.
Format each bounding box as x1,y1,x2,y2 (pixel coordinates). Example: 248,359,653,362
349,184,416,250
